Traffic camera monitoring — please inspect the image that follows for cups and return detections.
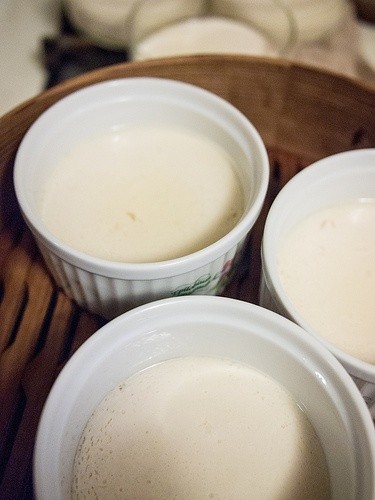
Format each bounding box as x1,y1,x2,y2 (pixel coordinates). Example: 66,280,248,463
258,149,375,402
13,76,270,317
124,0,300,71
34,296,375,500
